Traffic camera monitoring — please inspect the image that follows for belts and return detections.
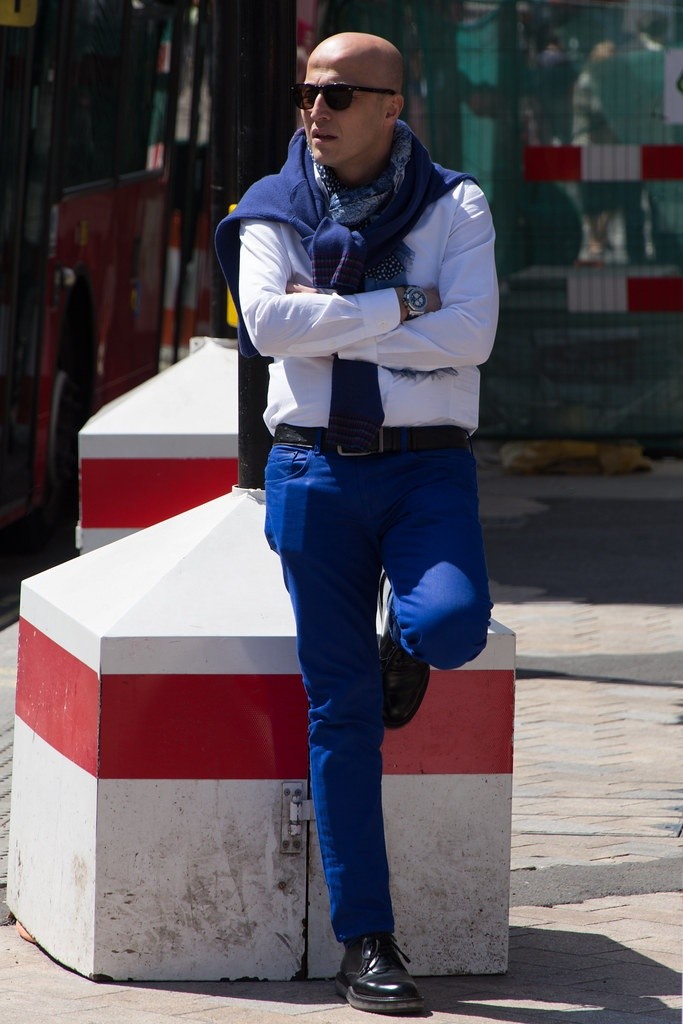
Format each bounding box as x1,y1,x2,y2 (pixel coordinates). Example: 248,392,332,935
273,423,470,456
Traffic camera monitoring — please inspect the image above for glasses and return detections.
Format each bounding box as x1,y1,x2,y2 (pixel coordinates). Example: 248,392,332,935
290,84,395,111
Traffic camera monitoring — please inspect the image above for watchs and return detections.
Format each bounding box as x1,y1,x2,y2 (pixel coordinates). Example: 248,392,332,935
398,283,427,322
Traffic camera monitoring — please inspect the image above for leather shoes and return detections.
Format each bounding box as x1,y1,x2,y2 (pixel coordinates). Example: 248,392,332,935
380,632,431,728
336,934,425,1013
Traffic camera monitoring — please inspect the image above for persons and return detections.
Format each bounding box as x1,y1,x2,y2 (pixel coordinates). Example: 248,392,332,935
536,14,683,273
215,33,501,1012
